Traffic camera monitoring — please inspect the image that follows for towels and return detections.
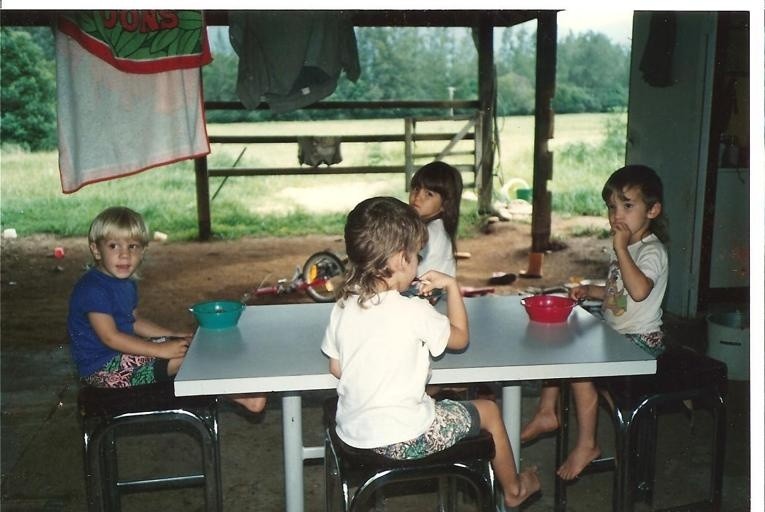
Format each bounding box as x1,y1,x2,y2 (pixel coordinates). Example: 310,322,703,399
48,11,213,194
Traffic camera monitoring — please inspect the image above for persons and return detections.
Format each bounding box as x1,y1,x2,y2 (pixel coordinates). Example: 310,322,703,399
318,195,542,508
520,162,673,481
64,206,269,416
406,160,463,280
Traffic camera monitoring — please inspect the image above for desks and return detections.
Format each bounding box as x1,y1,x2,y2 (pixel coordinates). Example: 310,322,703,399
174,298,658,511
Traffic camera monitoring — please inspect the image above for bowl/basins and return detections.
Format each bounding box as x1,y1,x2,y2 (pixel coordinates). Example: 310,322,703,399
189,301,245,330
519,295,579,323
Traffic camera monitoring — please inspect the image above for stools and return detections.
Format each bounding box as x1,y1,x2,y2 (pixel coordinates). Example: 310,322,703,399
78,383,222,511
556,350,726,512
324,391,496,510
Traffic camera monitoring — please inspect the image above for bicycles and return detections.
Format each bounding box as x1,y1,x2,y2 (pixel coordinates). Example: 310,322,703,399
249,252,346,299
